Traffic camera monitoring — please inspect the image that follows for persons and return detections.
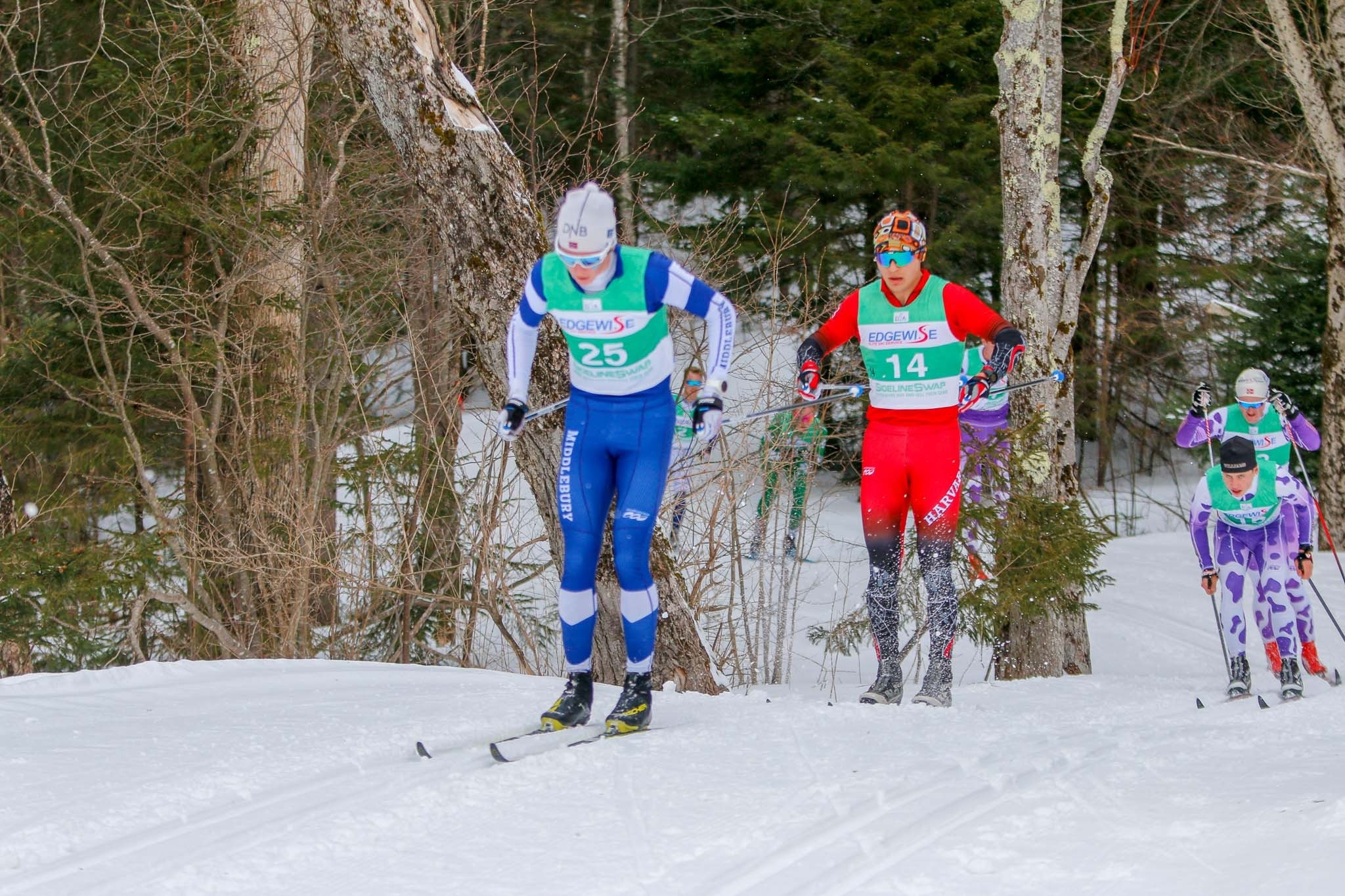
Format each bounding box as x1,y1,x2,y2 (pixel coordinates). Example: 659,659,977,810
666,366,706,548
1190,434,1315,701
746,393,830,563
791,209,1026,708
494,178,743,740
954,328,1009,584
1175,365,1329,677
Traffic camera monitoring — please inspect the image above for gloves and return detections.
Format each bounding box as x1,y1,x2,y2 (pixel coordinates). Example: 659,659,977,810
1269,388,1300,420
499,399,529,441
690,398,724,441
1190,381,1212,418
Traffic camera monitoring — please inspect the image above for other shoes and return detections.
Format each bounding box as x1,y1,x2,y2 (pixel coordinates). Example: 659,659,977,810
914,658,953,706
752,536,768,560
1281,657,1303,696
1227,655,1253,695
606,672,652,733
1301,641,1327,675
861,664,905,705
784,533,797,556
1264,641,1284,678
667,531,691,556
541,671,595,730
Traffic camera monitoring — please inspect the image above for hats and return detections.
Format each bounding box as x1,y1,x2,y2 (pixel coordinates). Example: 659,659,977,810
873,209,927,261
1219,436,1258,474
1235,367,1270,398
556,182,617,252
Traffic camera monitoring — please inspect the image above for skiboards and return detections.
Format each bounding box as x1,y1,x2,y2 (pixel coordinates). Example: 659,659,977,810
1196,656,1314,709
762,657,958,706
403,671,662,764
1262,641,1342,688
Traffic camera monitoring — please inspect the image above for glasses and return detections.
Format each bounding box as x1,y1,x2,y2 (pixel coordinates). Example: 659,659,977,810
684,380,703,386
793,412,815,422
875,245,926,267
1235,397,1269,409
555,236,613,268
